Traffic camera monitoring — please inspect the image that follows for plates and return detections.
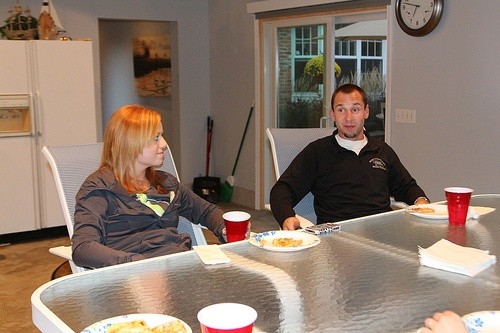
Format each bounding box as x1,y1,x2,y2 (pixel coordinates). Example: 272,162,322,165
461,311,500,332
248,229,321,252
80,312,193,333
406,204,449,219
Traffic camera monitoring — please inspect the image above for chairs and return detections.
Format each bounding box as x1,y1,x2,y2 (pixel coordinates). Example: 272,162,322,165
43,142,261,280
265,127,411,229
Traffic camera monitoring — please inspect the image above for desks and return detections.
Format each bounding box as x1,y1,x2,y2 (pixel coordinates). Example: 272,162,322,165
31,194,500,333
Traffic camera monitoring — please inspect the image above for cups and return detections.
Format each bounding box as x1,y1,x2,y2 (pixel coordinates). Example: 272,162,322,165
444,186,474,227
221,210,251,243
197,302,258,333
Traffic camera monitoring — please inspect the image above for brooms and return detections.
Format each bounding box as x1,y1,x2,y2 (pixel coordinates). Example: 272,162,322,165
220,106,254,204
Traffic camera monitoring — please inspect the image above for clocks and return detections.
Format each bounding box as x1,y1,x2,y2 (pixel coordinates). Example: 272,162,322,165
395,0,444,36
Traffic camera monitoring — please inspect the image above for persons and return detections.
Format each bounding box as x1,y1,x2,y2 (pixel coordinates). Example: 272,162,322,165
71,104,251,272
270,85,430,232
419,310,469,333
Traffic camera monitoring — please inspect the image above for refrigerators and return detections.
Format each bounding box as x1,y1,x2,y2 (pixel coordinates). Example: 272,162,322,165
0,39,98,235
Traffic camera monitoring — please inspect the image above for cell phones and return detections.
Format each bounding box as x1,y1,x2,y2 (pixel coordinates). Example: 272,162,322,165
304,223,340,235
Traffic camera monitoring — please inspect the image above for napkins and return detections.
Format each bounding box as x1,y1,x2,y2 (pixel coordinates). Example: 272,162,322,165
418,237,497,277
